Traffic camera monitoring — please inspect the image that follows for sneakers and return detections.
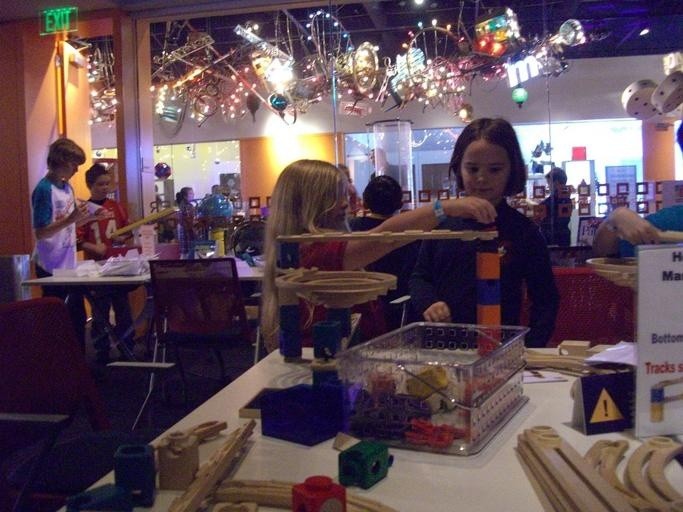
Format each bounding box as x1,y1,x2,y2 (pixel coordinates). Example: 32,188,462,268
93,337,137,353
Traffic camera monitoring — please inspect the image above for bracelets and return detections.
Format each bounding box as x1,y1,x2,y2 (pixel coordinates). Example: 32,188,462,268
432,199,446,223
604,218,616,233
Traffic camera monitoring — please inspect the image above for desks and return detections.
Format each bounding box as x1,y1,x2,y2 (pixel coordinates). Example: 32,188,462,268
57,346,683,512
20,264,266,401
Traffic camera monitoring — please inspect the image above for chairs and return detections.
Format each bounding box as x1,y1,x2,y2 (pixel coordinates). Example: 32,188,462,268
0,296,177,512
149,257,260,414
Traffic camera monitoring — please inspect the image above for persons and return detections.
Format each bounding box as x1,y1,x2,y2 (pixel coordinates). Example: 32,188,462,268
368,149,403,185
198,184,233,221
176,186,194,214
74,162,138,355
518,167,573,246
194,184,220,211
406,117,560,350
348,176,402,232
31,136,89,349
261,159,498,353
591,120,682,258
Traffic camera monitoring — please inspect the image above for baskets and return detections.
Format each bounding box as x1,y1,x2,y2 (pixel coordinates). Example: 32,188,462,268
338,320,531,454
